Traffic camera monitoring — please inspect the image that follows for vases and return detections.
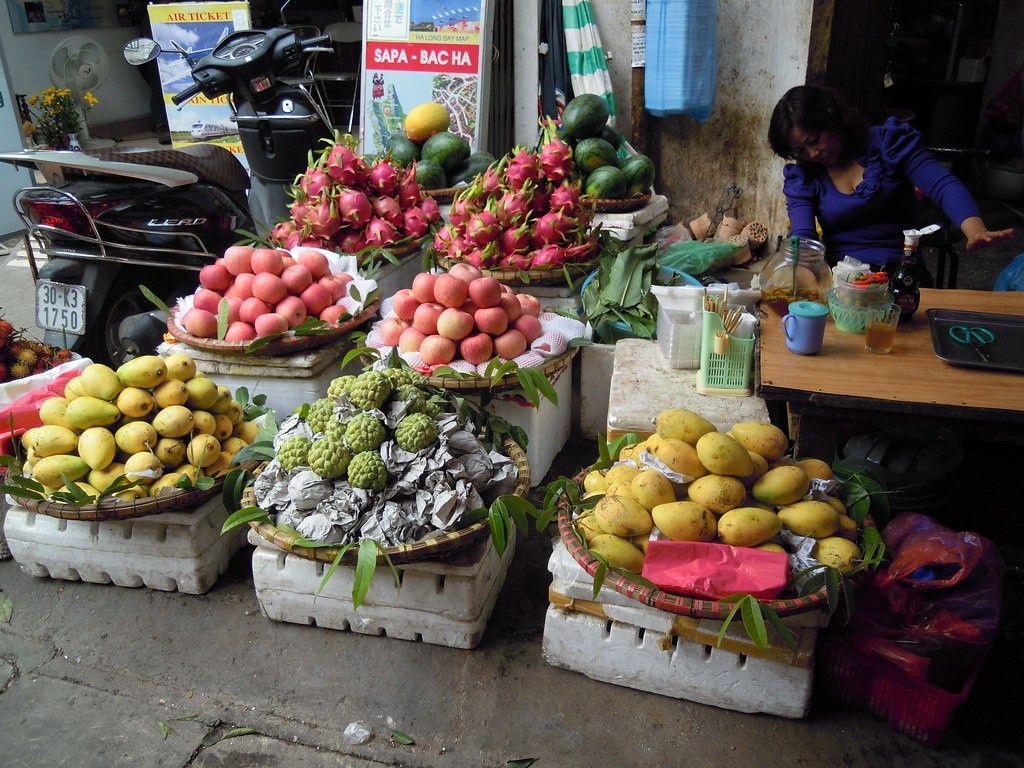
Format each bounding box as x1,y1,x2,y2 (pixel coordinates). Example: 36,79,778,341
66,132,80,151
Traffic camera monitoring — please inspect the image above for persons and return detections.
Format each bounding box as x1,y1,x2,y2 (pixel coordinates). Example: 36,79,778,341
373,73,384,100
768,86,1015,288
138,15,171,144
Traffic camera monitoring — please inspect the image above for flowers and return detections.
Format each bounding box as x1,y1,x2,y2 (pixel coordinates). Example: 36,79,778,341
26,87,100,138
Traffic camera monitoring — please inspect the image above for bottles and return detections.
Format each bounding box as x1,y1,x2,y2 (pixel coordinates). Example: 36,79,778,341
758,237,833,316
889,224,941,321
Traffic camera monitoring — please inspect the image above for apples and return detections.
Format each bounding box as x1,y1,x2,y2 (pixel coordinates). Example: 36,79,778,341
183,246,355,343
380,263,567,366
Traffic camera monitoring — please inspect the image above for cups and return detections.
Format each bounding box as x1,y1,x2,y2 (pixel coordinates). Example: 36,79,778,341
864,301,901,355
782,300,830,356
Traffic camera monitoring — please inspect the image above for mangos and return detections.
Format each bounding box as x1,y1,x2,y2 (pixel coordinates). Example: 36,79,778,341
576,410,861,578
21,356,259,502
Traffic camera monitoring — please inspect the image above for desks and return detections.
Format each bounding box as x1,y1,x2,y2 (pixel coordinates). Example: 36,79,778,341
754,273,1024,477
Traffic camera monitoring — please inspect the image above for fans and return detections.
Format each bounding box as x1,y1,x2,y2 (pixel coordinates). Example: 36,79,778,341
48,35,119,152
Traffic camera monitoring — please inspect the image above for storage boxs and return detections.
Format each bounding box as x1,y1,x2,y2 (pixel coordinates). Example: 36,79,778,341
0,251,995,739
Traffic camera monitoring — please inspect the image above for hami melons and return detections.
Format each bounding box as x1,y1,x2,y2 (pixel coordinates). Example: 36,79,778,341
403,102,450,140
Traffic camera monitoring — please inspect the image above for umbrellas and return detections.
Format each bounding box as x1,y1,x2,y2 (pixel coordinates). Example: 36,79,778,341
536,1,618,131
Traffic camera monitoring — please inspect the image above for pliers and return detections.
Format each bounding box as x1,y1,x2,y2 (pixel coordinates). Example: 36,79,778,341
702,187,741,243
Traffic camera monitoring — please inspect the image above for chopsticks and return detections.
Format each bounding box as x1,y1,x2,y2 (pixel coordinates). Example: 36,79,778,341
703,287,743,335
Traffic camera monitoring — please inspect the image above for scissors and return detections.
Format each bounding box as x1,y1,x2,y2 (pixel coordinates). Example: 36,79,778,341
950,324,995,363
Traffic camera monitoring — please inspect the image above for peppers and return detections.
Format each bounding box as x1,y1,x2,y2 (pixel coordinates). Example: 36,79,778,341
842,264,889,288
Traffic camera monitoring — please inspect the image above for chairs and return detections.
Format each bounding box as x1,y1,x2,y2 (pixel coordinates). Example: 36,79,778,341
276,21,362,133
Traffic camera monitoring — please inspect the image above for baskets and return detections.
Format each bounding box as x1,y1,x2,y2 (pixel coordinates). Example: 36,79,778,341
696,294,756,396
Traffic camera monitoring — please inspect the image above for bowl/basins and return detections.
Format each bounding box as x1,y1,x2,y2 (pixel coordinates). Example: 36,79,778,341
828,288,894,332
650,285,761,370
836,278,889,306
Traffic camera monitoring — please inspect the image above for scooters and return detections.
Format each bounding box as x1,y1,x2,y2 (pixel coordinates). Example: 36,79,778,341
4,1,335,371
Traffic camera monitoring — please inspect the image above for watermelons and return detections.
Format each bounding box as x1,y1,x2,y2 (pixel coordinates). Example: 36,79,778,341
361,94,655,200
